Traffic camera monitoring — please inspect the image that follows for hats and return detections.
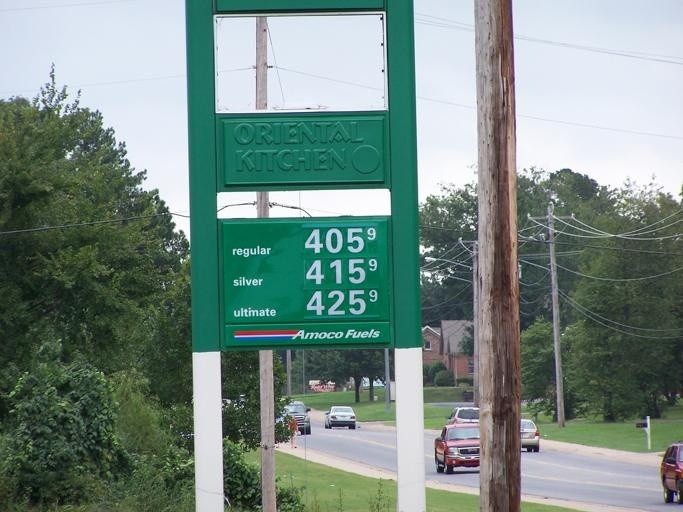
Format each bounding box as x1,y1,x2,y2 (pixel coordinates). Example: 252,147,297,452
520,419,540,453
434,406,481,474
324,405,356,429
660,442,683,504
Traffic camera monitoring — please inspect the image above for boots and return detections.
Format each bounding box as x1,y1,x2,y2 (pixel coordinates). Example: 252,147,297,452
275,400,311,435
